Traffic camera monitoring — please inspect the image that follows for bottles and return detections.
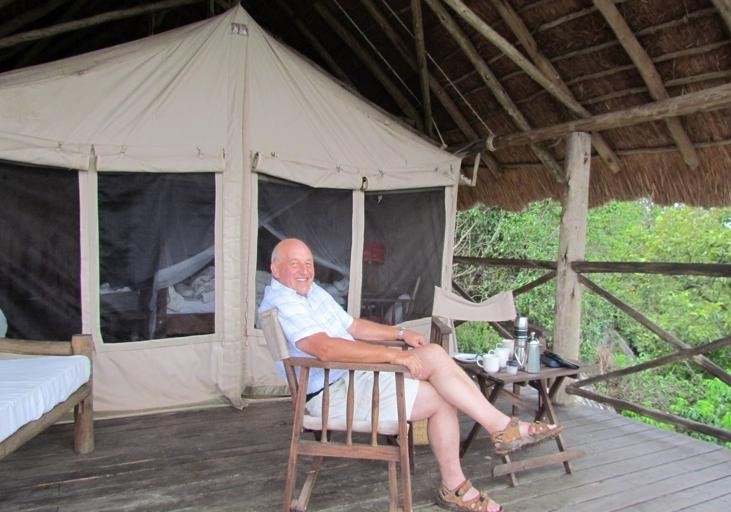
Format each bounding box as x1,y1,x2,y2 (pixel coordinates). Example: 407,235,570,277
526,331,541,374
513,316,529,371
505,360,518,375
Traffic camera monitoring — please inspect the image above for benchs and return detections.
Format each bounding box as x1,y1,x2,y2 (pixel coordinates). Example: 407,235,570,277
0,334,95,463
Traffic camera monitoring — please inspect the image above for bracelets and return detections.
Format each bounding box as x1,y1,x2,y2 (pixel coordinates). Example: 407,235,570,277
398,325,405,340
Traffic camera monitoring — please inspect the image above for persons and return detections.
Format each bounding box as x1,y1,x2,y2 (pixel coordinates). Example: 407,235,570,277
258,238,563,512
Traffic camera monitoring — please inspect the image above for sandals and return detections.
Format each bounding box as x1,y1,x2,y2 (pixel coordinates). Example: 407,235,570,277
435,479,504,512
491,415,563,456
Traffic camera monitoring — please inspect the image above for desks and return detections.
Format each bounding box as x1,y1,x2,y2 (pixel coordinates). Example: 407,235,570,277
451,351,583,489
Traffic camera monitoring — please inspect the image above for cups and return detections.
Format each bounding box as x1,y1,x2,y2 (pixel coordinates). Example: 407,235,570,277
476,354,500,374
487,347,509,369
495,338,514,363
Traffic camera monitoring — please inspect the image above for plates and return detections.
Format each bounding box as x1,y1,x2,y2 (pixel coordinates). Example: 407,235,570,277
453,353,483,364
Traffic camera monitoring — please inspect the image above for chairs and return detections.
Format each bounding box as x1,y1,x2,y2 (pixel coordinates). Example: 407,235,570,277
257,307,452,512
